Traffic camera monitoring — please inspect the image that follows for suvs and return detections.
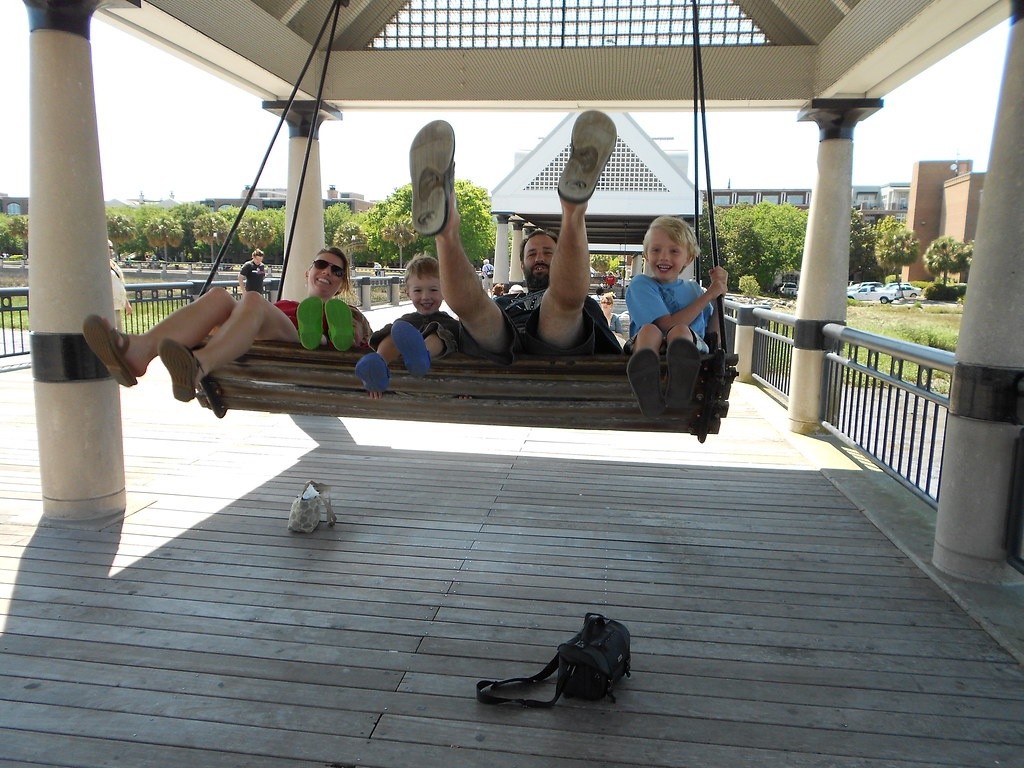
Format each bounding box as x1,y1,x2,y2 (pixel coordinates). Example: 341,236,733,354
777,282,797,295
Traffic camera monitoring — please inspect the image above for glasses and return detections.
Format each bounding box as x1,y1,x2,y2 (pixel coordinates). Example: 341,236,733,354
109,246,114,250
255,254,263,257
312,259,346,277
601,302,611,305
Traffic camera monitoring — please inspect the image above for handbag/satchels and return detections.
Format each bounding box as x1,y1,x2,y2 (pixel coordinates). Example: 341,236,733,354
476,612,631,709
287,480,338,533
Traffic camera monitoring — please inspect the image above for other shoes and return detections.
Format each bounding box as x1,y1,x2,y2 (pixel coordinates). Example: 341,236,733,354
297,296,323,349
324,298,354,351
355,353,391,392
391,320,432,379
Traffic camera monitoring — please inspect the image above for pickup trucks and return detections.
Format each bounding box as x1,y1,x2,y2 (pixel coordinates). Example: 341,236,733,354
847,286,898,304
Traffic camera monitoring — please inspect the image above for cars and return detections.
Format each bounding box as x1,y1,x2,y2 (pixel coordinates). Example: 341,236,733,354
884,283,922,298
848,280,884,291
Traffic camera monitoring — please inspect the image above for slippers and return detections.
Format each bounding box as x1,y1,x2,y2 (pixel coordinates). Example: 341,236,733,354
83,314,137,388
159,338,203,402
558,110,617,204
408,120,455,236
664,337,701,413
627,348,666,418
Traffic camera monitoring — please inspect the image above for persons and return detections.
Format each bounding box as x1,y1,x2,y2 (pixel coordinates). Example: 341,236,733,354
374,262,383,276
355,254,459,399
82,238,372,417
624,216,730,420
409,110,622,369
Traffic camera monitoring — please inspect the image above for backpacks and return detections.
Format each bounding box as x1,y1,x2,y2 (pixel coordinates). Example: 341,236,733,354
485,266,493,278
